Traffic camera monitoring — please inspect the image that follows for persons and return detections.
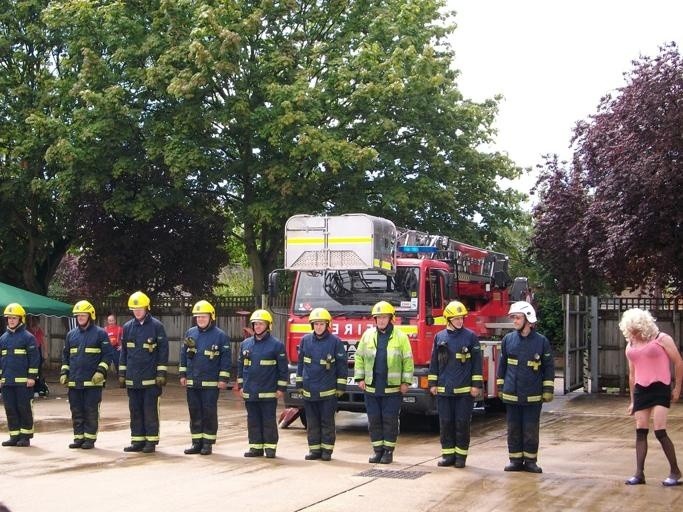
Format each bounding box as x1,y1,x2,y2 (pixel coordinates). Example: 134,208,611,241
119,291,169,453
354,301,414,464
618,308,683,487
0,303,41,446
428,300,483,468
104,314,122,375
179,299,232,454
27,316,50,397
236,309,288,458
296,308,348,461
60,301,113,449
496,301,554,473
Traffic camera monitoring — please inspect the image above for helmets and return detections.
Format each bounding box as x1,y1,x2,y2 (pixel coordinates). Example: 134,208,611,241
309,307,332,328
4,303,26,323
191,300,216,321
73,300,96,321
371,301,396,322
127,290,151,310
443,300,467,319
507,301,537,323
249,309,273,331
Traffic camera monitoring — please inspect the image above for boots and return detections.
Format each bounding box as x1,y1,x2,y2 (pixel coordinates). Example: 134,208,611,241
16,437,30,446
82,440,94,449
265,448,276,458
524,462,542,473
184,442,201,454
201,444,212,454
123,443,145,452
70,440,84,448
245,449,263,457
505,462,523,471
380,449,393,463
321,450,331,461
369,451,383,462
2,437,19,446
438,455,455,466
143,443,155,452
305,451,321,460
455,458,466,466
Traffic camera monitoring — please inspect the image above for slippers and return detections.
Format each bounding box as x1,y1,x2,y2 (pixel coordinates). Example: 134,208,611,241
625,476,645,484
663,477,682,486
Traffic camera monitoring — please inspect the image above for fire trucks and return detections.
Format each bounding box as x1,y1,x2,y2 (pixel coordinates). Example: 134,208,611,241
267,210,536,430
